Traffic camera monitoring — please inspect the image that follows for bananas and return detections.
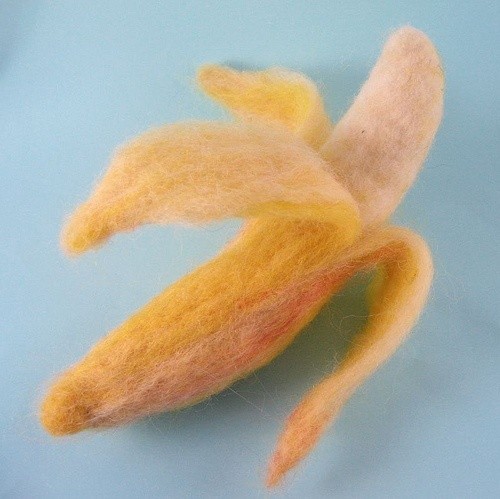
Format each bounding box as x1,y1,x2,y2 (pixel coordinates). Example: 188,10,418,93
37,25,446,489
